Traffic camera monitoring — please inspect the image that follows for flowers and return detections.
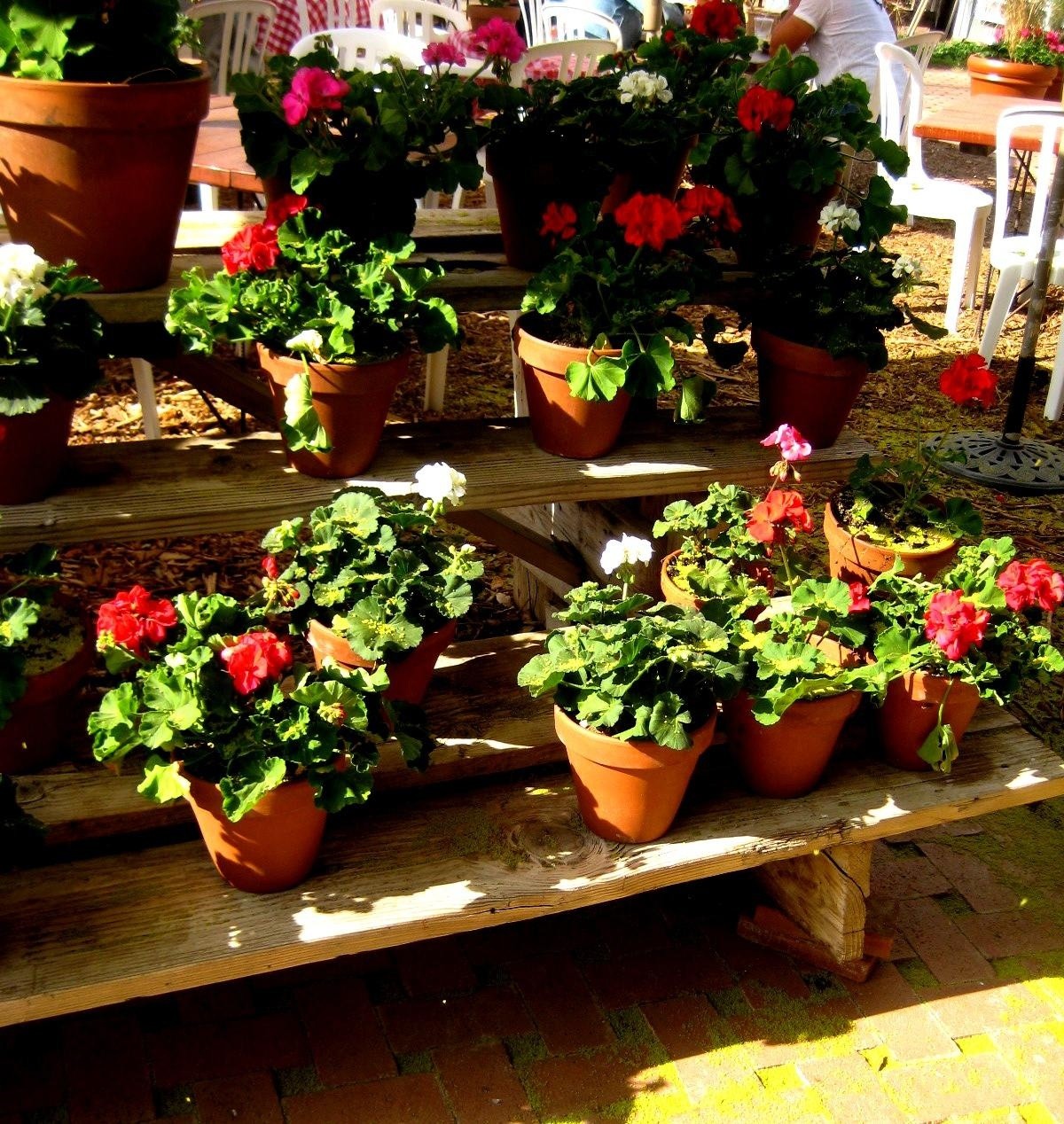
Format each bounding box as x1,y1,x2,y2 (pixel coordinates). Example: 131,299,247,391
0,460,485,825
514,350,1064,776
1,0,950,451
973,21,1064,68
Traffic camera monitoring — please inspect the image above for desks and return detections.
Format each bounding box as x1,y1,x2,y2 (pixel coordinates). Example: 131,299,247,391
911,90,1063,155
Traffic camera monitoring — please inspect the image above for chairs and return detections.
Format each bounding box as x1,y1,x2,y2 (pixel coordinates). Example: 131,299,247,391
182,0,279,96
977,105,1063,423
508,40,617,86
724,10,782,74
292,1,370,34
541,5,624,53
370,1,471,43
895,29,943,79
289,27,434,74
872,44,995,336
519,1,553,48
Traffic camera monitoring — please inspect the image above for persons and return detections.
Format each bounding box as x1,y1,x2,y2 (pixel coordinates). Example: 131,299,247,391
244,0,743,121
767,0,894,111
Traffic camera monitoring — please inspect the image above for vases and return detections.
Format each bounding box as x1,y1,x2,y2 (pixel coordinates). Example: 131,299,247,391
182,766,331,894
967,52,1064,101
23,631,88,720
254,342,412,478
866,650,982,771
509,318,641,457
746,325,872,450
0,385,78,487
0,58,211,294
303,612,452,706
728,178,839,281
593,112,702,222
481,146,620,267
251,151,422,245
551,703,717,846
731,639,863,792
